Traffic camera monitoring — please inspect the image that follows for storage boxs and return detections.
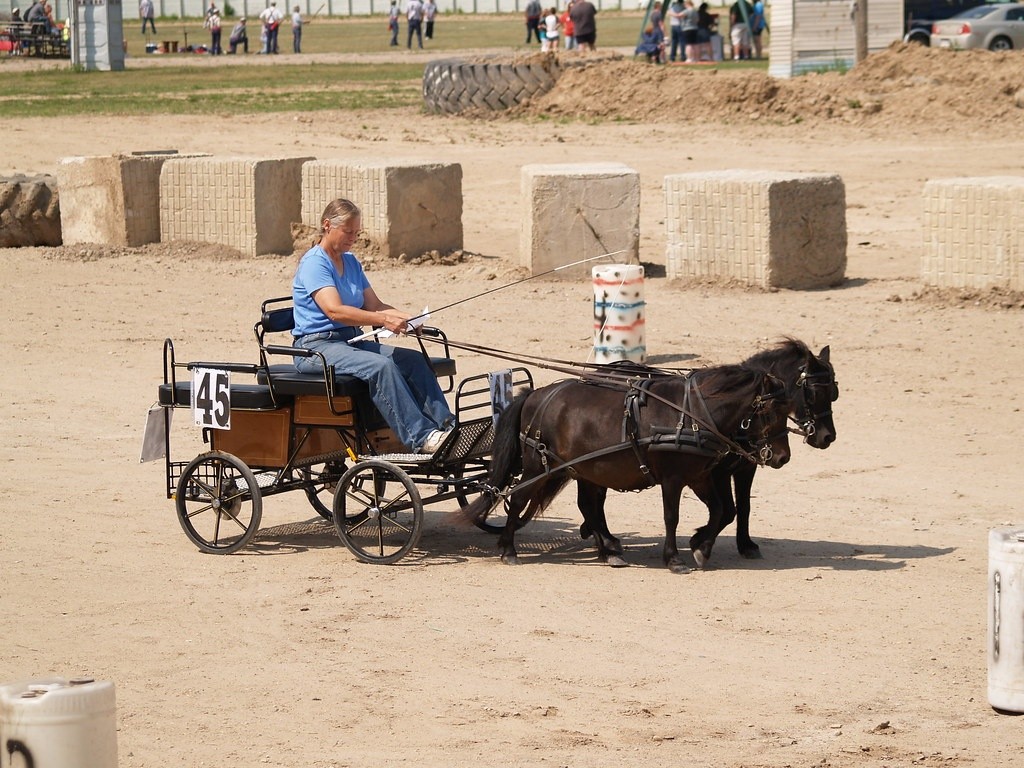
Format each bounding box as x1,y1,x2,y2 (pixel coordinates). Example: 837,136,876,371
145,43,156,52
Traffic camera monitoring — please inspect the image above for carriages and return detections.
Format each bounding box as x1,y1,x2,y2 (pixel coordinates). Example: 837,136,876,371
139,294,837,567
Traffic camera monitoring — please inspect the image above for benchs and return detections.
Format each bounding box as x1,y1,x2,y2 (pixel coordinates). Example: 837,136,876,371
158,338,289,411
0,21,67,58
253,296,456,432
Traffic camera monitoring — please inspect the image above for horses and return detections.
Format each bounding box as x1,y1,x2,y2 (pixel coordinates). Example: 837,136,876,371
577,336,840,559
442,365,791,574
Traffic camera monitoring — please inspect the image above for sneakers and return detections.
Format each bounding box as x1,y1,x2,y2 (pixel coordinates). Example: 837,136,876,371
418,429,452,453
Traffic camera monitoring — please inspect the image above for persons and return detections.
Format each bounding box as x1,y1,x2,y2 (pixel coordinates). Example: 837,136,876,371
63,18,71,53
421,0,438,40
290,199,460,454
634,0,770,64
10,7,23,56
223,17,249,55
138,0,157,35
23,0,58,37
259,0,284,55
388,0,399,47
553,0,598,54
404,0,424,50
524,0,560,53
203,2,222,55
290,5,311,53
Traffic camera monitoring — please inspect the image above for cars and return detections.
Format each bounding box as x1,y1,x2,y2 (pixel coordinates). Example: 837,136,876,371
930,2,1024,51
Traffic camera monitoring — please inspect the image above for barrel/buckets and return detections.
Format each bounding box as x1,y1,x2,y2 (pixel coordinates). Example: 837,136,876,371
590,263,647,365
986,525,1024,715
0,676,117,768
